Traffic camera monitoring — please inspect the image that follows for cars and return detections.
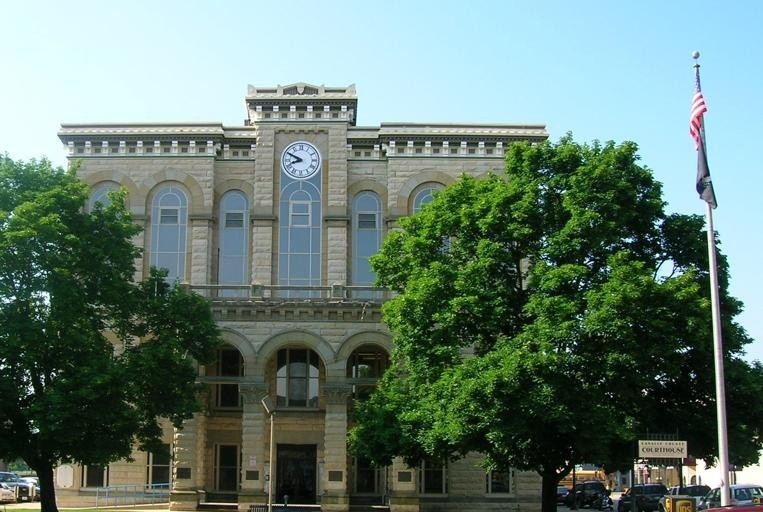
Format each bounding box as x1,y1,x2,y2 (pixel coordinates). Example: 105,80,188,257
556,459,763,512
0,471,41,503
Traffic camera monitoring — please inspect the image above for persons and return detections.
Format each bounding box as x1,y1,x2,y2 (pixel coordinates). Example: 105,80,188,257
281,479,290,508
605,480,612,491
592,471,600,480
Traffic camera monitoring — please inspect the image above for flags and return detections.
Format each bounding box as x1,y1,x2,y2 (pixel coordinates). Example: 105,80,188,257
691,64,717,210
